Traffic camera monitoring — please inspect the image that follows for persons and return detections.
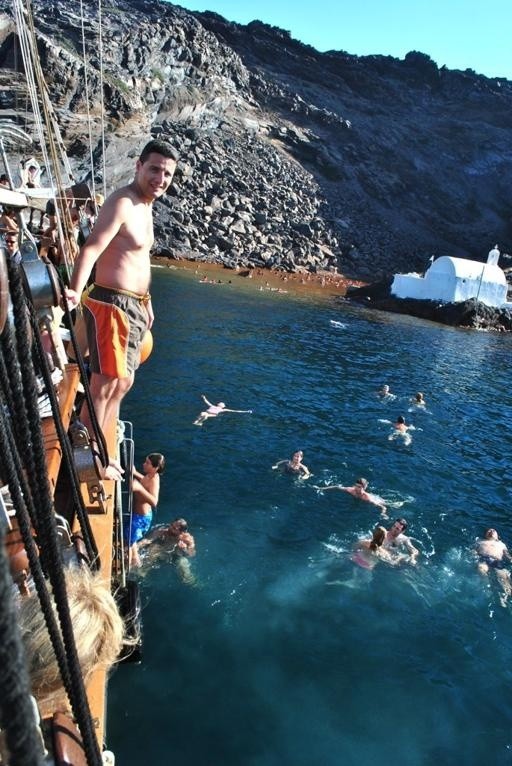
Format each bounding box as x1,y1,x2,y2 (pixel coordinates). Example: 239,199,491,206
471,526,512,609
0,138,198,720
191,394,253,426
272,446,418,574
374,385,427,448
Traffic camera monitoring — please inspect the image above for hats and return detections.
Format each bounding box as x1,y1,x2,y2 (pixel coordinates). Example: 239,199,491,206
96,193,105,207
